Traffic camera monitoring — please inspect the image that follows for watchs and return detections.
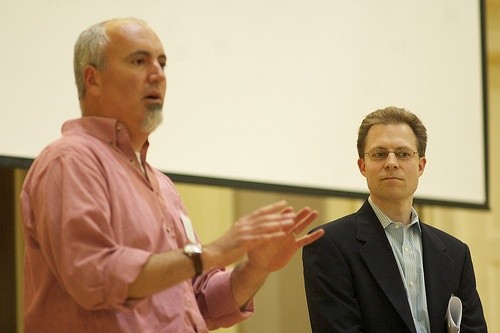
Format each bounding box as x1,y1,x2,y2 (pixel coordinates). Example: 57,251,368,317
184,242,203,277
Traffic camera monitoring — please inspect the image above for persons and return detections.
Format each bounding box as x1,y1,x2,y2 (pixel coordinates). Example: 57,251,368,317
301,106,488,333
20,17,324,333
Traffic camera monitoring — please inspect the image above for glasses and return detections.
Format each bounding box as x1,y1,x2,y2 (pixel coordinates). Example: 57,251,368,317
363,148,420,160
